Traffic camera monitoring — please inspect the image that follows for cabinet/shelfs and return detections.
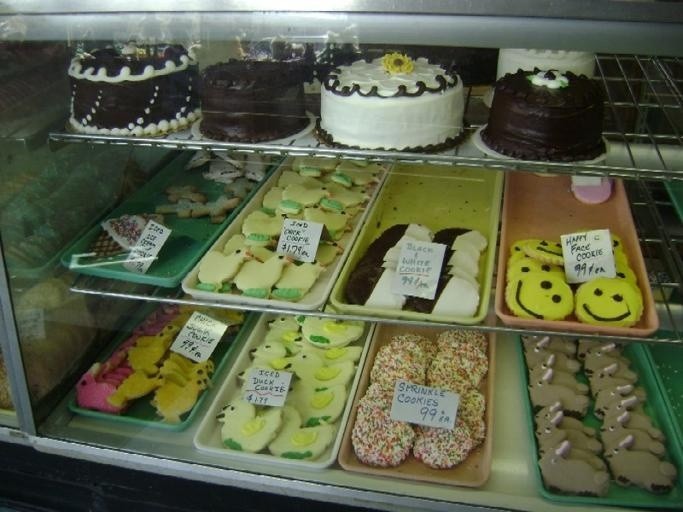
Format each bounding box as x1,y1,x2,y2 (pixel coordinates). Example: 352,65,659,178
0,1,683,512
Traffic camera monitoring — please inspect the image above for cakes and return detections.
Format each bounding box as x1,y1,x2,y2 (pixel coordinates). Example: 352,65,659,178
198,58,308,139
68,39,201,132
76,303,245,425
481,65,606,164
521,332,677,496
570,171,612,204
185,151,266,182
345,225,488,316
145,177,254,227
216,315,364,461
318,52,468,152
488,46,596,106
349,329,488,472
505,228,645,328
196,160,387,300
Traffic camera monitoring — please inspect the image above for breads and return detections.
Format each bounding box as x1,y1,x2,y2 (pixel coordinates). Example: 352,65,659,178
1,277,96,411
0,155,133,268
